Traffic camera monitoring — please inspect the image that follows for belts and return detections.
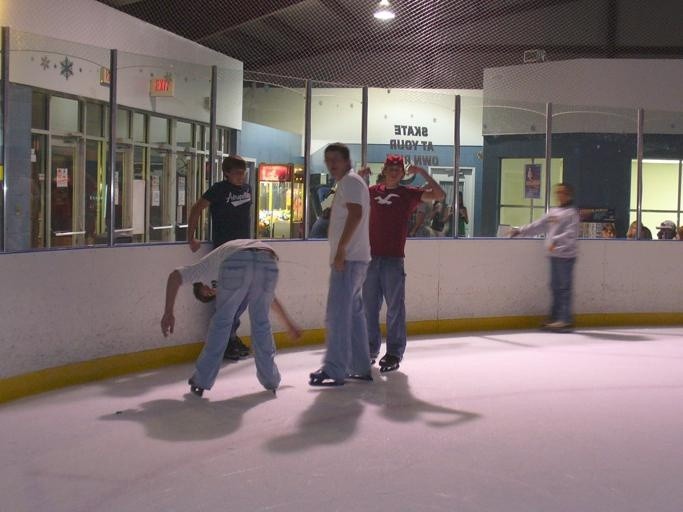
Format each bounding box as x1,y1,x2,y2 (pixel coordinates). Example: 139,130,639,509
242,247,276,254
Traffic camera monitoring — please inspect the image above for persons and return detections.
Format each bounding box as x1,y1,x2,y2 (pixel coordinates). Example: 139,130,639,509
161,239,282,393
639,227,652,240
309,181,469,238
510,184,579,330
600,223,614,238
656,220,677,239
625,221,642,239
675,225,682,240
356,154,447,368
188,154,254,359
306,142,375,381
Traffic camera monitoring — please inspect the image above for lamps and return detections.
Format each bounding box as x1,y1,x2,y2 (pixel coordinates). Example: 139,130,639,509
373,0,395,19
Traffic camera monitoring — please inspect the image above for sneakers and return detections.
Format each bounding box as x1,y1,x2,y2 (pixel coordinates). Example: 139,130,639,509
310,369,331,380
223,337,251,359
379,352,401,367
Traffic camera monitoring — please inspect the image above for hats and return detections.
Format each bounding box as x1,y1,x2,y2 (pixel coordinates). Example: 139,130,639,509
384,153,403,166
656,220,676,229
